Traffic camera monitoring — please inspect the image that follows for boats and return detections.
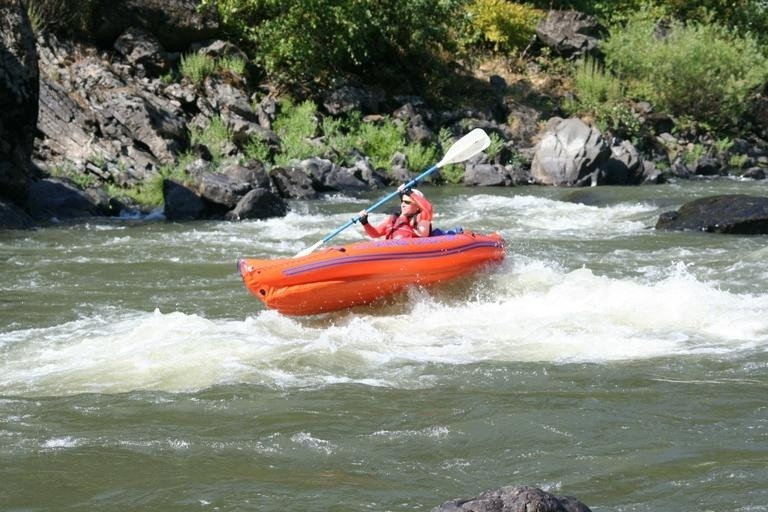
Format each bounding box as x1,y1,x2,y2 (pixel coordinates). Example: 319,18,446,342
237,230,508,316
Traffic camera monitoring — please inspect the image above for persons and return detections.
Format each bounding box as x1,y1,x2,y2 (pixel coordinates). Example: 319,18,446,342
358,184,434,240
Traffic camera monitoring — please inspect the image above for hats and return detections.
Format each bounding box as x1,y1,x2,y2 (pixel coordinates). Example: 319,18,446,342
399,188,424,199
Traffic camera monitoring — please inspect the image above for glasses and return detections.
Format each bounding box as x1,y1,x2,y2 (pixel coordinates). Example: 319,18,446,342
401,200,412,204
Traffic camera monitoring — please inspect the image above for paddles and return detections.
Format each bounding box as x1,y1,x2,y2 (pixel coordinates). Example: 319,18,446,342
292,127,490,259
